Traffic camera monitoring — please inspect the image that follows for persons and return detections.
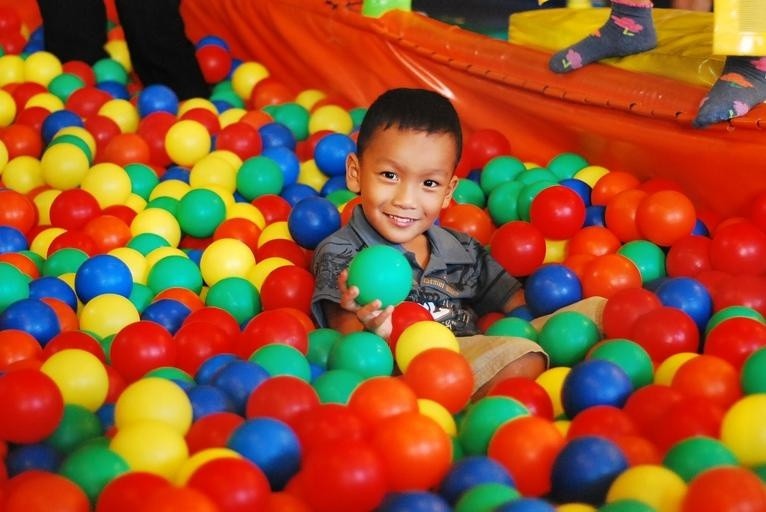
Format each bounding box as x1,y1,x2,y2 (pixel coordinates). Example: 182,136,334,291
550,0,766,128
37,0,209,99
